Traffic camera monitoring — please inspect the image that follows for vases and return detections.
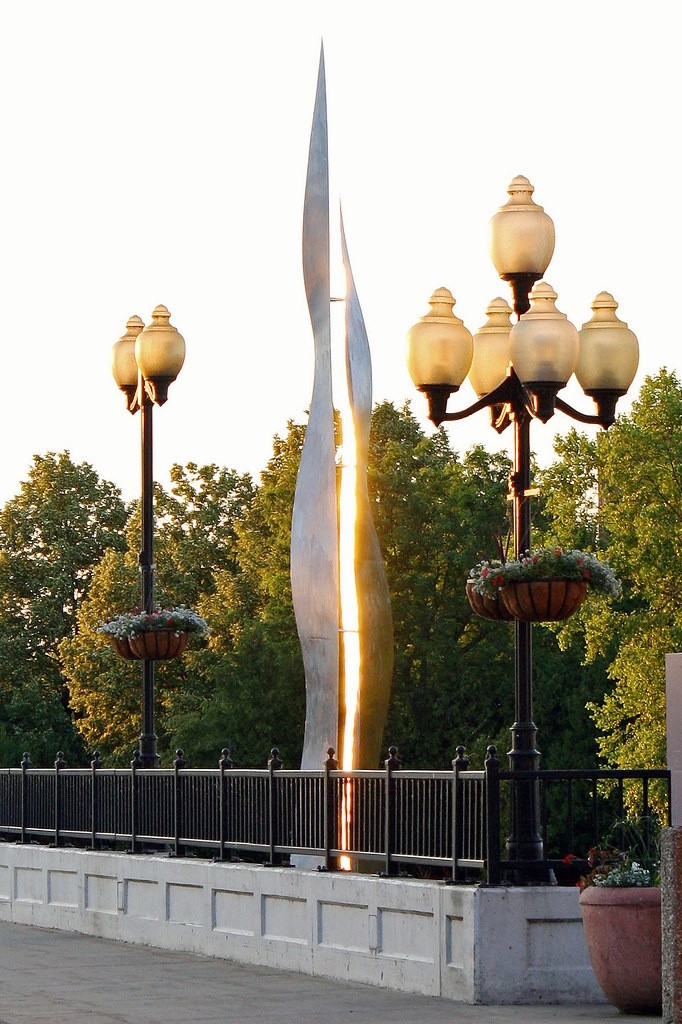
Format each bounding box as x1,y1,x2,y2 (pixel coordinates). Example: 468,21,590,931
500,576,586,621
109,632,140,661
578,886,660,1015
126,628,189,661
465,579,515,623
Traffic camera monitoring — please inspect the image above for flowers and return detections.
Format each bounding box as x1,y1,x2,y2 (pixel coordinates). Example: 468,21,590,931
89,613,145,634
467,558,504,580
113,606,210,638
471,543,622,610
560,811,662,894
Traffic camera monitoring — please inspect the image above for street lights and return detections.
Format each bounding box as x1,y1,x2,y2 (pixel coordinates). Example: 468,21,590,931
112,299,174,769
400,173,646,887
